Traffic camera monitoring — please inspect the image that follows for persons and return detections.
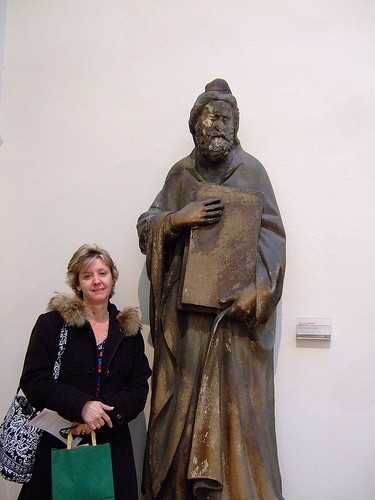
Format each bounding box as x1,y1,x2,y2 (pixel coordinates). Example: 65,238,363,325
15,242,153,500
136,78,290,500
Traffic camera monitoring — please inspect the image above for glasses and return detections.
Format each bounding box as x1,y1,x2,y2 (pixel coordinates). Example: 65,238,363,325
56,425,92,442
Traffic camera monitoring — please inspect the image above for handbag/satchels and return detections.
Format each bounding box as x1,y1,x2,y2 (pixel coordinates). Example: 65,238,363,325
51,428,117,499
2,324,68,481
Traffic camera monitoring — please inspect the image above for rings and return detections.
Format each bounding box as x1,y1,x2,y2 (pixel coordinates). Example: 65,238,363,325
99,419,104,424
94,421,99,427
82,431,86,436
